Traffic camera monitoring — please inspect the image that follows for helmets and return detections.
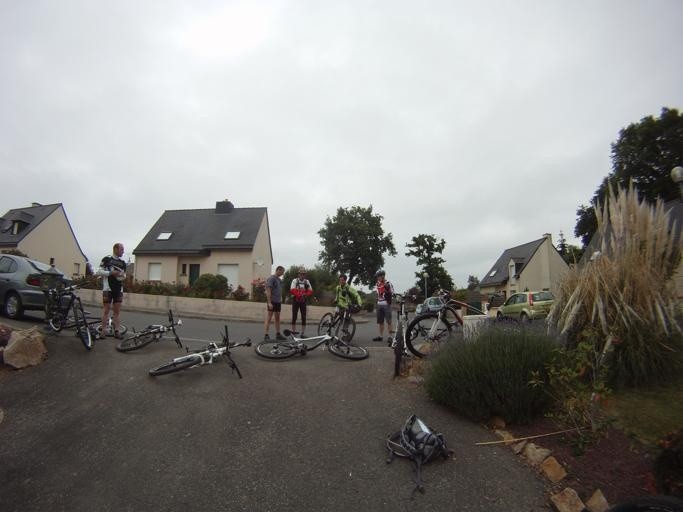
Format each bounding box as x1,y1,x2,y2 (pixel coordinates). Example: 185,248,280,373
375,270,384,275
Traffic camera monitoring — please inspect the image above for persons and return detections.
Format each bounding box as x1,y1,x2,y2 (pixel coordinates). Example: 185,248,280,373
289,268,313,338
94,240,129,341
371,269,394,342
330,274,362,341
259,264,287,342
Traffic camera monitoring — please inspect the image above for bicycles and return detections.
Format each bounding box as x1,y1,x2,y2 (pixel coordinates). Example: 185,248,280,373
41,276,102,349
387,292,416,375
317,302,362,343
44,310,127,338
148,325,253,379
406,285,526,361
117,309,183,351
255,329,369,361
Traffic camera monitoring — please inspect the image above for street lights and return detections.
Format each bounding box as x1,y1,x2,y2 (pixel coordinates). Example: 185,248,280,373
423,272,429,300
670,166,683,200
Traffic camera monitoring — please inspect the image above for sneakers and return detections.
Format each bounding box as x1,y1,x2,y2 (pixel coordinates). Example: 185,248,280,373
373,336,383,341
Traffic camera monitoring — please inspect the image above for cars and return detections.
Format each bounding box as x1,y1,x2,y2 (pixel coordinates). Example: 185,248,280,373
497,290,556,324
415,304,424,316
0,253,72,320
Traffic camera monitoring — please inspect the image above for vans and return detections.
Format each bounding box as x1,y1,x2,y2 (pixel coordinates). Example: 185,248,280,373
423,297,444,313
